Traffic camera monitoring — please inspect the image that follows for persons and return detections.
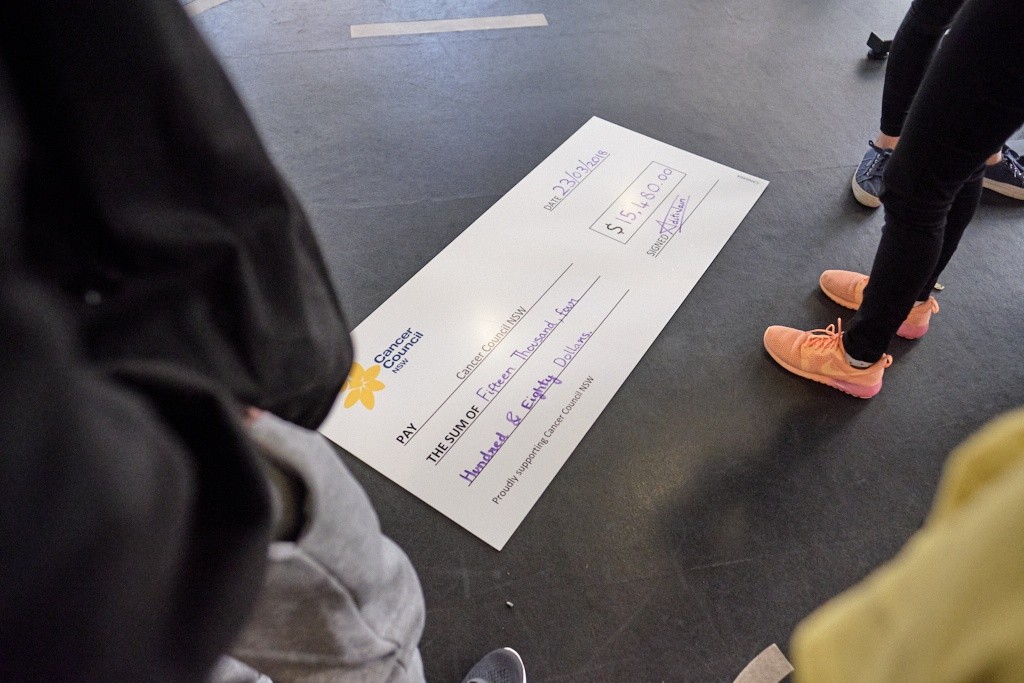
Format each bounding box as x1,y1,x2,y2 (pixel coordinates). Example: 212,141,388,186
763,0,1024,683
0,0,528,683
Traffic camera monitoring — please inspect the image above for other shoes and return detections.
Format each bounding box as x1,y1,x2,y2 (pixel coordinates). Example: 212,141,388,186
462,646,526,683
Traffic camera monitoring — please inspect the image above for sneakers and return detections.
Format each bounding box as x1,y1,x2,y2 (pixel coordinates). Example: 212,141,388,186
851,138,895,207
763,318,892,399
820,269,939,339
982,143,1024,201
